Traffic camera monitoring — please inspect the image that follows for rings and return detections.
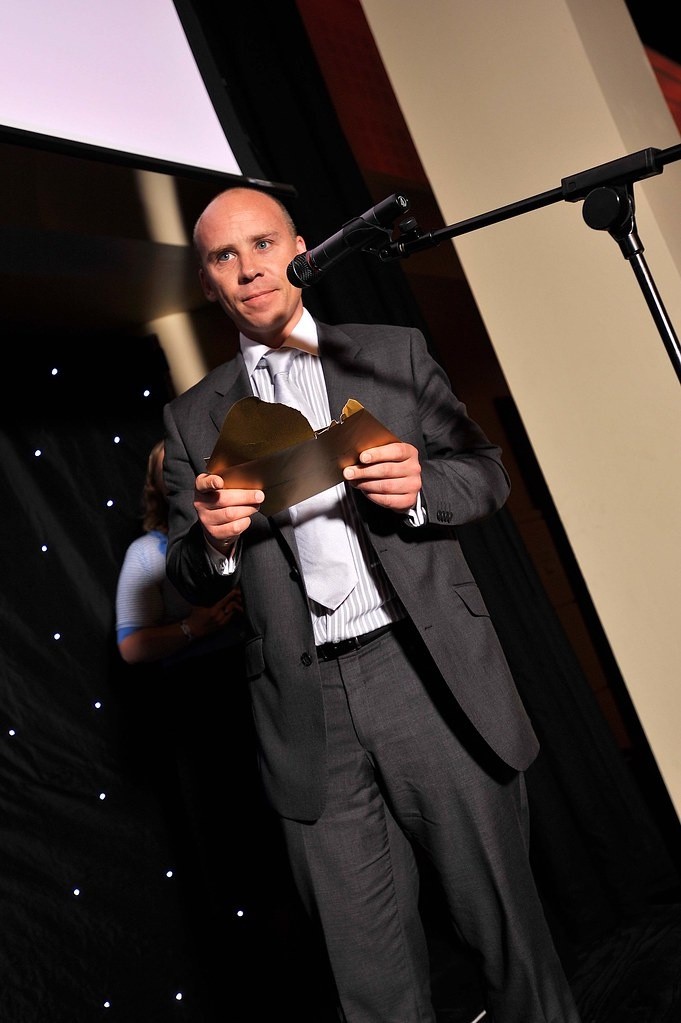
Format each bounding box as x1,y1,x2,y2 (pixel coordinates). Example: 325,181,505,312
224,609,229,615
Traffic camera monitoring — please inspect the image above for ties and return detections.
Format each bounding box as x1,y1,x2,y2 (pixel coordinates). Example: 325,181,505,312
257,347,357,609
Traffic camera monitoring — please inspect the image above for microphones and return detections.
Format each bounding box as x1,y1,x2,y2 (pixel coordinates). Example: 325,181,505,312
286,191,412,289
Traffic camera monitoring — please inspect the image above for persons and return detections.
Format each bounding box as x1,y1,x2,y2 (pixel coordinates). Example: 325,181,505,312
116,436,245,675
157,188,582,1023
